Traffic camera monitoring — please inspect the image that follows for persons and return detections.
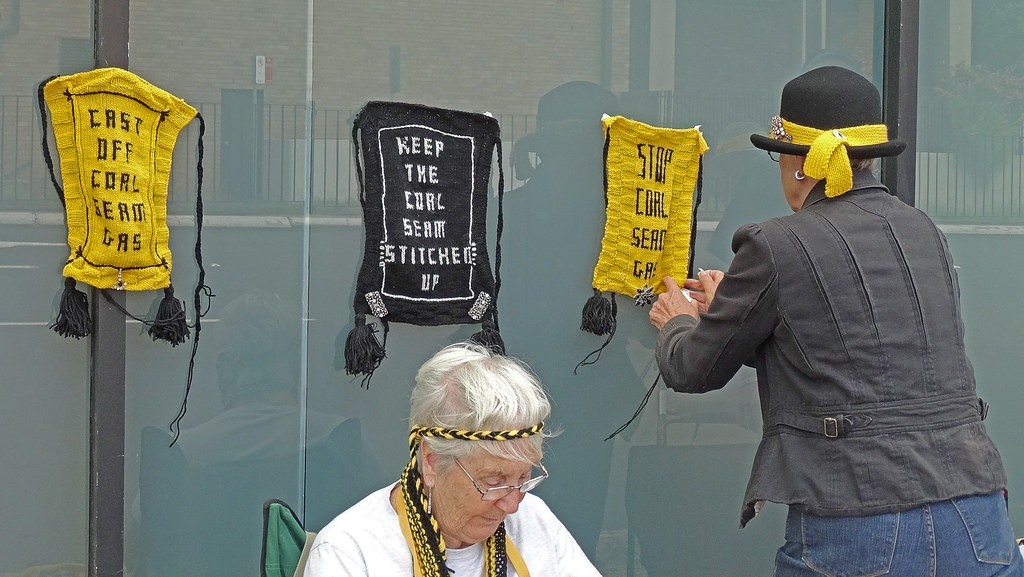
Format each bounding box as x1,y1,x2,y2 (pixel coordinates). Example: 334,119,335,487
302,341,605,577
648,63,1024,577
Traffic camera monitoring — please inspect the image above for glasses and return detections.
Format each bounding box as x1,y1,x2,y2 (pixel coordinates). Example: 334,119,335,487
766,150,780,162
452,457,548,501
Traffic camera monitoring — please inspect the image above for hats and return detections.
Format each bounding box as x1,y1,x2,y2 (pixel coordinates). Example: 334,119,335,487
750,66,906,199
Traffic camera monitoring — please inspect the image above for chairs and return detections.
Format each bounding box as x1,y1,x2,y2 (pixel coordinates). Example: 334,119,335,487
259,498,317,577
